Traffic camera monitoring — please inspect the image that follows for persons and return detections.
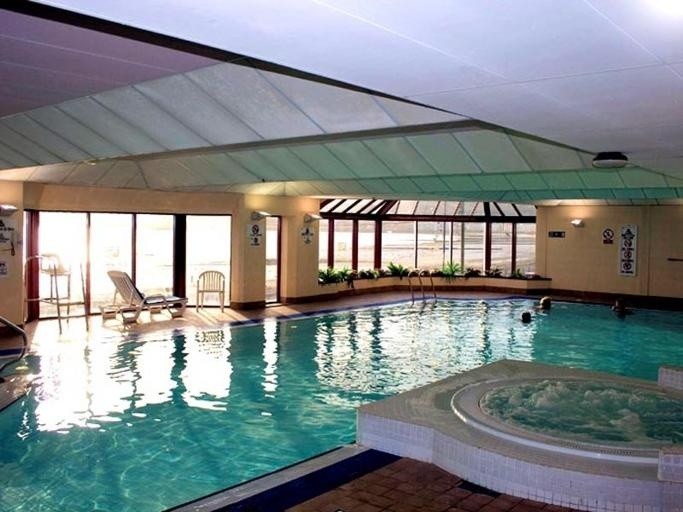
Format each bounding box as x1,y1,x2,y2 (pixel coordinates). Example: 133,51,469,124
521,310,532,322
536,296,552,311
610,296,626,311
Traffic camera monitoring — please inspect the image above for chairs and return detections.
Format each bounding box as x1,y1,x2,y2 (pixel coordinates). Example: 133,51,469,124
99,270,226,327
